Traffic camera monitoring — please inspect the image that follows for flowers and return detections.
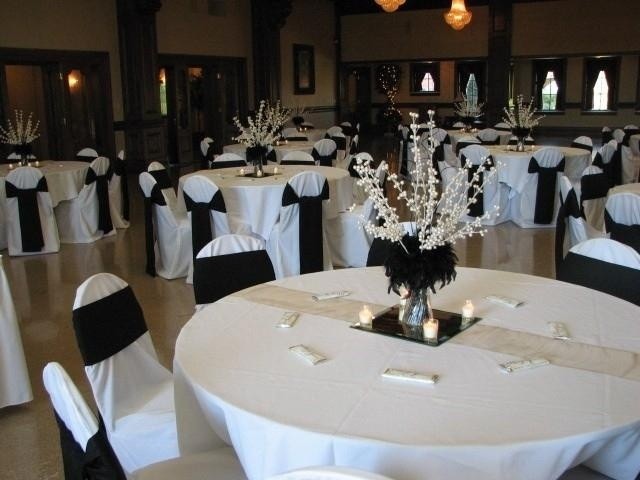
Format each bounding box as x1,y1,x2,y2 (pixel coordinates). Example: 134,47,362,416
345,109,506,291
259,101,290,136
501,95,546,138
289,103,313,129
454,95,486,119
374,64,403,130
231,101,282,162
2,108,44,150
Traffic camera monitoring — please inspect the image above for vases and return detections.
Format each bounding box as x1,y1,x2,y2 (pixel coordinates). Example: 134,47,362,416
402,277,434,329
464,120,472,132
516,136,524,150
251,150,263,178
16,141,30,166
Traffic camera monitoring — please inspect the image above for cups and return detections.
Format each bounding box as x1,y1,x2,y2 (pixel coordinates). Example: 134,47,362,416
238,167,245,176
460,298,476,322
422,318,439,346
272,166,279,174
359,302,374,329
256,170,263,177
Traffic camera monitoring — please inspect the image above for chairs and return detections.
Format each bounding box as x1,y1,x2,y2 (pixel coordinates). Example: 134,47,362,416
41,356,248,480
383,158,389,191
4,151,40,163
74,146,99,161
345,149,374,178
398,120,404,140
300,121,316,130
354,123,359,136
624,124,639,136
507,133,533,145
577,164,611,231
453,120,467,129
105,149,132,229
333,133,349,159
600,125,612,144
618,132,640,180
492,120,513,130
603,191,640,255
593,140,618,180
419,120,436,130
614,129,629,144
348,131,360,155
455,134,480,159
145,160,181,213
553,175,609,281
328,161,383,268
281,150,314,166
138,171,193,282
363,220,426,267
396,125,429,181
341,120,351,130
74,272,181,467
558,237,640,308
198,143,220,160
4,166,63,256
210,153,248,169
193,232,278,309
308,138,338,166
472,127,501,146
425,125,458,168
323,125,343,139
202,136,218,152
287,131,308,144
571,135,592,151
265,144,278,162
53,156,116,243
459,144,513,225
433,136,470,217
507,147,565,228
182,175,249,283
265,169,333,279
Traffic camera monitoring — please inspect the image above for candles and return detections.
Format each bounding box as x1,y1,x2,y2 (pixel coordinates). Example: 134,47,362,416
607,179,640,202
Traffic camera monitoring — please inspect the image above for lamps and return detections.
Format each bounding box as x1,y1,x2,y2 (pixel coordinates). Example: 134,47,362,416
377,0,405,11
442,0,473,31
